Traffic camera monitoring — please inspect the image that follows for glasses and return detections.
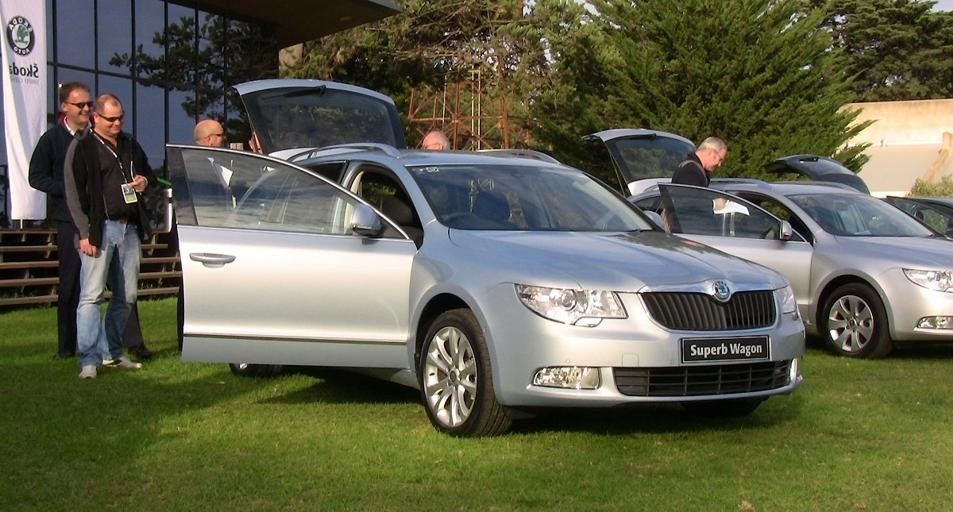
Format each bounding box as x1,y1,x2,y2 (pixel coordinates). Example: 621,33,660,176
208,132,224,136
713,148,723,161
63,101,93,108
423,143,442,149
96,110,125,122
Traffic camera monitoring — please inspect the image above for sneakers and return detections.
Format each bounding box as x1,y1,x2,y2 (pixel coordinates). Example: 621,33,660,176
78,364,97,379
101,355,142,369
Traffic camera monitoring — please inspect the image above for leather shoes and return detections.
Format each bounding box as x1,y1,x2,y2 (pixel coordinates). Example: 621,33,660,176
128,346,153,362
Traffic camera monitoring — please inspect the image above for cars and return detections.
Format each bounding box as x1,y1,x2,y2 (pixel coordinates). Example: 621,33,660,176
761,153,953,241
163,77,807,439
579,127,953,361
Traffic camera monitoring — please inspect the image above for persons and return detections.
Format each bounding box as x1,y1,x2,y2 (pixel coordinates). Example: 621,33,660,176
422,131,451,151
28,83,153,360
662,137,727,236
65,93,154,379
176,120,233,353
